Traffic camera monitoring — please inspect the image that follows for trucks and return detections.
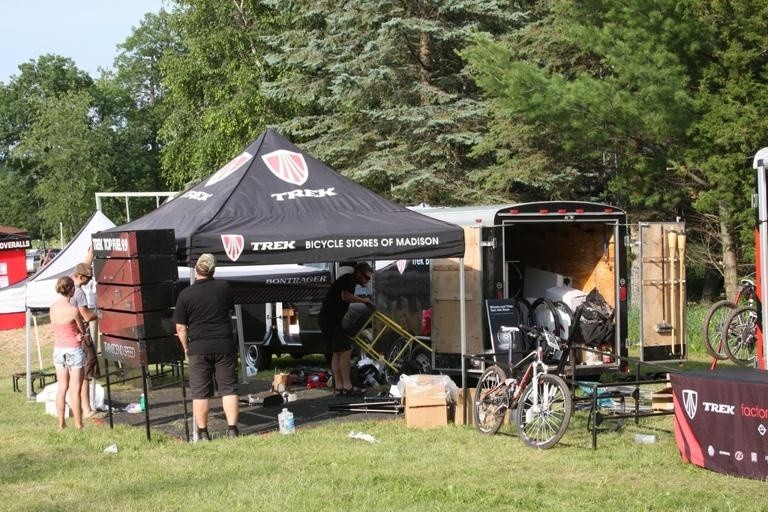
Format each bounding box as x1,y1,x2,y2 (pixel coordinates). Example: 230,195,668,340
236,200,685,375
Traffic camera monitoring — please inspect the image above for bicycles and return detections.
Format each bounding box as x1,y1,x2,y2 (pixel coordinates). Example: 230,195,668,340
473,324,573,449
703,279,762,365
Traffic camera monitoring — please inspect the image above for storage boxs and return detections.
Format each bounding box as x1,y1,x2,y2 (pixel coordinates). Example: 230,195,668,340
402,374,510,430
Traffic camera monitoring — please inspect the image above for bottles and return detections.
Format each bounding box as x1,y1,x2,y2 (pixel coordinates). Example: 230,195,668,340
277,407,295,435
139,392,145,411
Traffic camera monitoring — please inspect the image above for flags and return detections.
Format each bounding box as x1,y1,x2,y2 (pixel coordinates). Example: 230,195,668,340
666,371,767,481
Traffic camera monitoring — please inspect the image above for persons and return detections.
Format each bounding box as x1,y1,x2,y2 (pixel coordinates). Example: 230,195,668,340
315,262,374,397
173,252,241,446
48,274,88,433
69,243,103,420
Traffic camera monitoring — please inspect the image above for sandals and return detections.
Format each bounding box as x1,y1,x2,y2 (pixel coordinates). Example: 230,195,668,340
333,386,367,396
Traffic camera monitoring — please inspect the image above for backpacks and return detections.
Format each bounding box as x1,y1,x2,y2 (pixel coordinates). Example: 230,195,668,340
575,288,618,347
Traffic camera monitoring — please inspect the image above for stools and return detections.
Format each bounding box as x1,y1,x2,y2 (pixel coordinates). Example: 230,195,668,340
341,301,433,373
12,371,56,397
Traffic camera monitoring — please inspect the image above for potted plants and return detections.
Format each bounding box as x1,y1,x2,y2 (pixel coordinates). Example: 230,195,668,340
270,355,294,392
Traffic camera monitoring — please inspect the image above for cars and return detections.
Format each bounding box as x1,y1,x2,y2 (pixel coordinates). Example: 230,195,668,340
26,248,60,273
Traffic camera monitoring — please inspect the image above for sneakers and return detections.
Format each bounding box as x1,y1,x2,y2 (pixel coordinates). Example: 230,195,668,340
227,424,238,439
197,428,210,440
84,411,109,420
